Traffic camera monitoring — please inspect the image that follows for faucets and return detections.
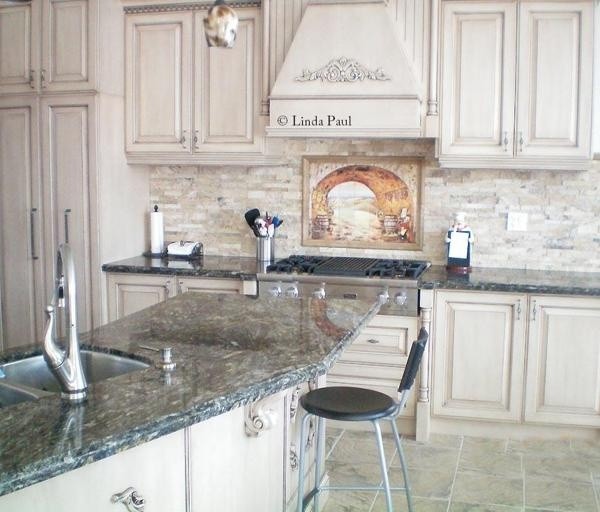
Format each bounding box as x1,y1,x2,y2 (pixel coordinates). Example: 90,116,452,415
42,243,88,391
52,400,88,466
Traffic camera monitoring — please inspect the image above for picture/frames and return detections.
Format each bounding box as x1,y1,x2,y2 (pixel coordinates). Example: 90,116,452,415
302,154,423,250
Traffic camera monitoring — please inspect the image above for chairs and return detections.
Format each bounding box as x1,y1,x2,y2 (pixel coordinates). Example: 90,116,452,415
295,324,429,512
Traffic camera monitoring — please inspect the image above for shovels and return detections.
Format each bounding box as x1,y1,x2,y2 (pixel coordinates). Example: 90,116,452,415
245,209,260,230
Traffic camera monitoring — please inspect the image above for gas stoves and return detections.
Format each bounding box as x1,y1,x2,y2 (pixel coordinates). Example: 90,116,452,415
256,255,431,316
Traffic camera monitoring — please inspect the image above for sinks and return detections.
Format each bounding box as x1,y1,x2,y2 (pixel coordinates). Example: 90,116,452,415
0,343,154,400
0,383,40,418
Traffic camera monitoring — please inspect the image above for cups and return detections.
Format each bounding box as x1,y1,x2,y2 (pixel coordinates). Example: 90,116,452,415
257,237,274,261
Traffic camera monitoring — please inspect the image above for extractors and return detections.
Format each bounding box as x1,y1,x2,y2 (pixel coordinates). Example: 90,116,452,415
266,0,425,137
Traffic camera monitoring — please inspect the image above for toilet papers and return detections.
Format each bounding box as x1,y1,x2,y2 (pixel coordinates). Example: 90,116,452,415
150,212,166,254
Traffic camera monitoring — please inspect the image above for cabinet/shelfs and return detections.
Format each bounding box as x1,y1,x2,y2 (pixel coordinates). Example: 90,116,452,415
327,314,415,421
439,0,593,167
123,7,274,158
104,273,245,323
0,93,152,352
434,289,600,428
0,1,124,92
